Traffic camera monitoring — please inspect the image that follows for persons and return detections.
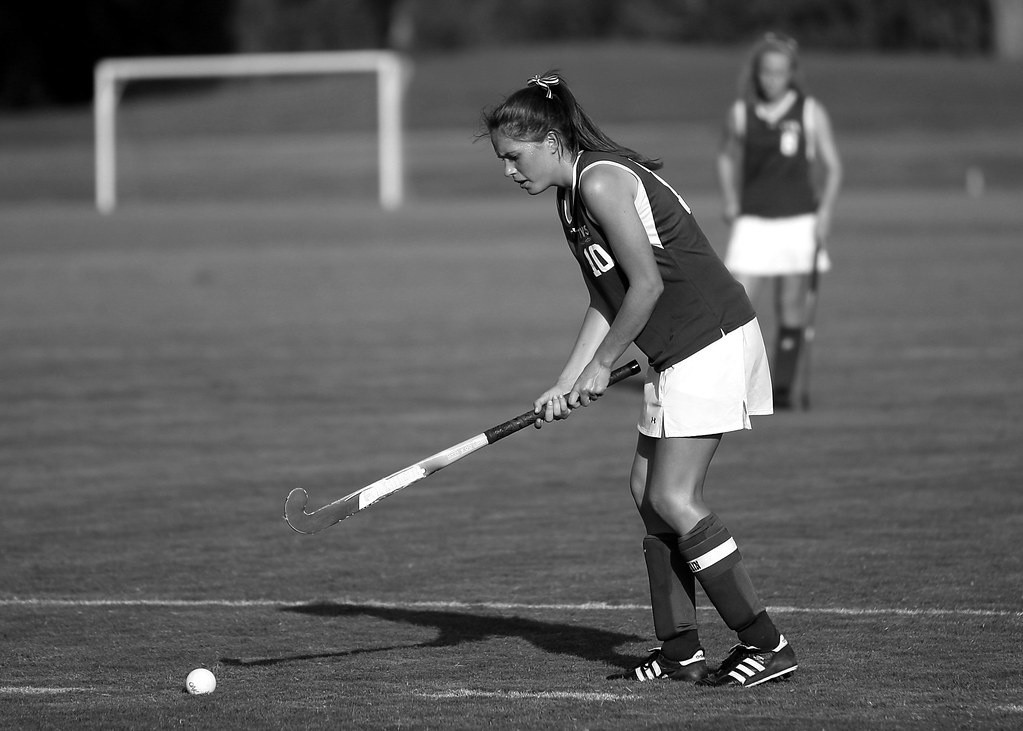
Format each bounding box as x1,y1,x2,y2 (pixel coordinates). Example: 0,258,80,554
714,32,844,409
483,70,798,688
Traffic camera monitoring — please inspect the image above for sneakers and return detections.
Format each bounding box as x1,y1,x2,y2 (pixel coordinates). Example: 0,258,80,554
695,633,798,688
606,646,708,684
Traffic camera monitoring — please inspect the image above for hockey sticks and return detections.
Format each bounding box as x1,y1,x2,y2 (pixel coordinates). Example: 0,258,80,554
796,244,819,406
284,358,642,538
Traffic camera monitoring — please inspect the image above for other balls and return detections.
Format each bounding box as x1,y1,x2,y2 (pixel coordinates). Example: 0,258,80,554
185,666,220,699
